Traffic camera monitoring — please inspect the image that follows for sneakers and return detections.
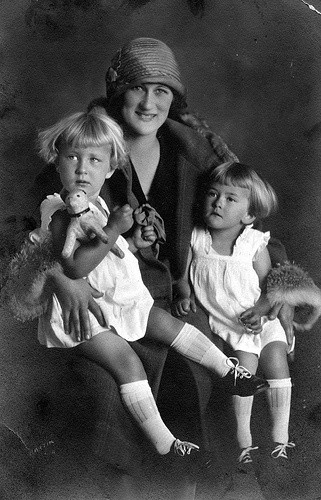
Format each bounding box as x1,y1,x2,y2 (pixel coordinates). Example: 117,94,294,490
270,442,296,469
219,356,270,397
161,439,214,473
237,444,259,474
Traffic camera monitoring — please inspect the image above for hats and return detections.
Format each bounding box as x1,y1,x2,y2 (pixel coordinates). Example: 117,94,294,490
105,37,186,101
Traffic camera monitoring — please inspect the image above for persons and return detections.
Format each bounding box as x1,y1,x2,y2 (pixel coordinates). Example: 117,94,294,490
28,110,270,470
0,37,321,500
171,160,296,474
